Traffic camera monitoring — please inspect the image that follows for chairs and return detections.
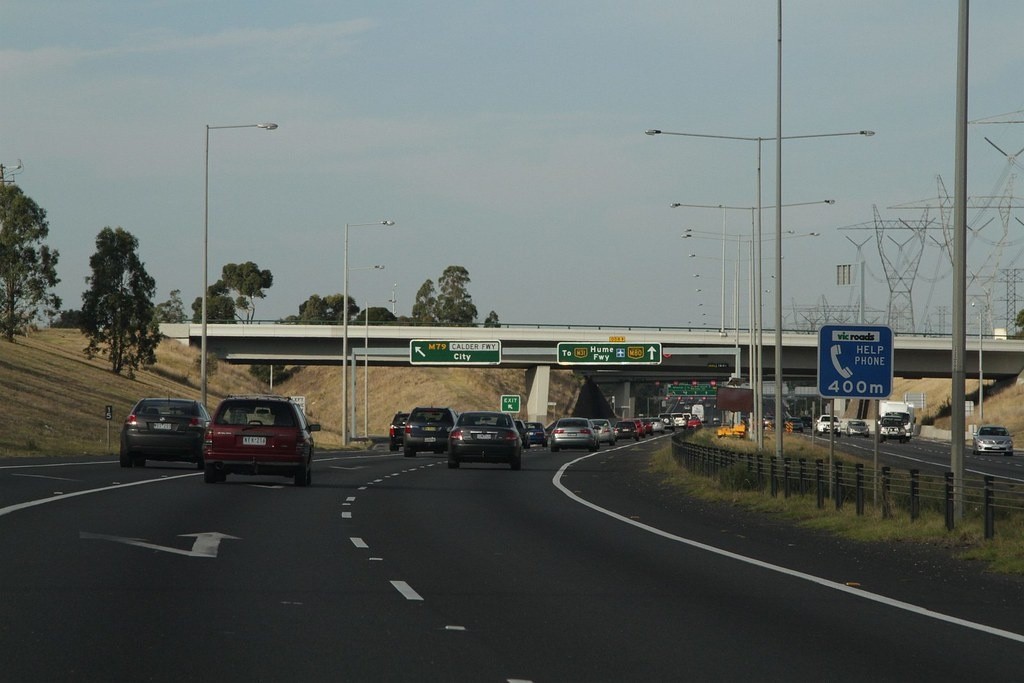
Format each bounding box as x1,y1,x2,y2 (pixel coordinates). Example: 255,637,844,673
147,408,159,414
496,418,506,426
274,407,292,425
463,418,475,426
229,408,247,424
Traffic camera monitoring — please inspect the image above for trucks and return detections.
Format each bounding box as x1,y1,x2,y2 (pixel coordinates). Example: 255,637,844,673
879,400,917,442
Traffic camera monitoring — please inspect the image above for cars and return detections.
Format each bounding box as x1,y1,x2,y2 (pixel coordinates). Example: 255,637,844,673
764,416,775,427
117,396,211,471
783,416,812,433
845,420,871,437
515,408,701,452
201,393,322,487
972,423,1015,456
445,410,530,471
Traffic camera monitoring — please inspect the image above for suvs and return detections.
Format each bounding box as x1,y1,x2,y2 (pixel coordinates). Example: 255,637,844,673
388,410,412,451
815,413,844,438
402,405,459,456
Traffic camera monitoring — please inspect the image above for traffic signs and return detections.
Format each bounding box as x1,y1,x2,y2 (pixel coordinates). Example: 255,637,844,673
409,339,502,366
500,395,521,414
555,340,663,367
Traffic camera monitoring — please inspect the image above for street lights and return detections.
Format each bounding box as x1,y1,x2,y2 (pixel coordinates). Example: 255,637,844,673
971,303,984,424
200,121,280,407
716,203,726,331
342,217,396,448
680,232,822,438
669,199,837,443
643,128,878,450
363,298,395,439
804,397,808,416
688,252,784,333
684,227,796,423
